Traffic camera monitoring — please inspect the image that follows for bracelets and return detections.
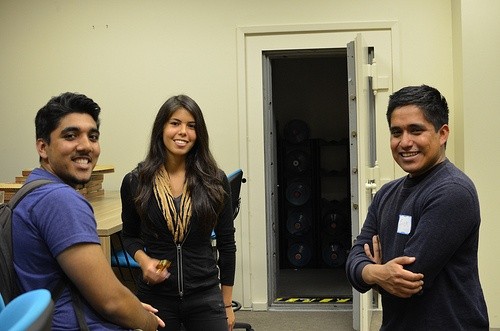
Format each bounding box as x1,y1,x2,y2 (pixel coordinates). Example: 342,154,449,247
225,304,232,307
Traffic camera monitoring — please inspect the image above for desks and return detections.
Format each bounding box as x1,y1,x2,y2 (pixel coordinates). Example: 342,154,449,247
87,190,123,266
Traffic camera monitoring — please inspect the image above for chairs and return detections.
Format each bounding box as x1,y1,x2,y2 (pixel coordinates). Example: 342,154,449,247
0,169,254,331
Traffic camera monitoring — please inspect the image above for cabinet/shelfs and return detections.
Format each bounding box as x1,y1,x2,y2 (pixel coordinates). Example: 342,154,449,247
278,139,351,269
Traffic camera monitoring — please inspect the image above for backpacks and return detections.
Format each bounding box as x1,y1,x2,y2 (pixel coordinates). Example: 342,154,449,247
0,179,89,331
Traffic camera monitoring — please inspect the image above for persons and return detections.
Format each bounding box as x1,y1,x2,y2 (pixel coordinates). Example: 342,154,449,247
121,95,236,331
346,86,490,331
8,92,164,331
